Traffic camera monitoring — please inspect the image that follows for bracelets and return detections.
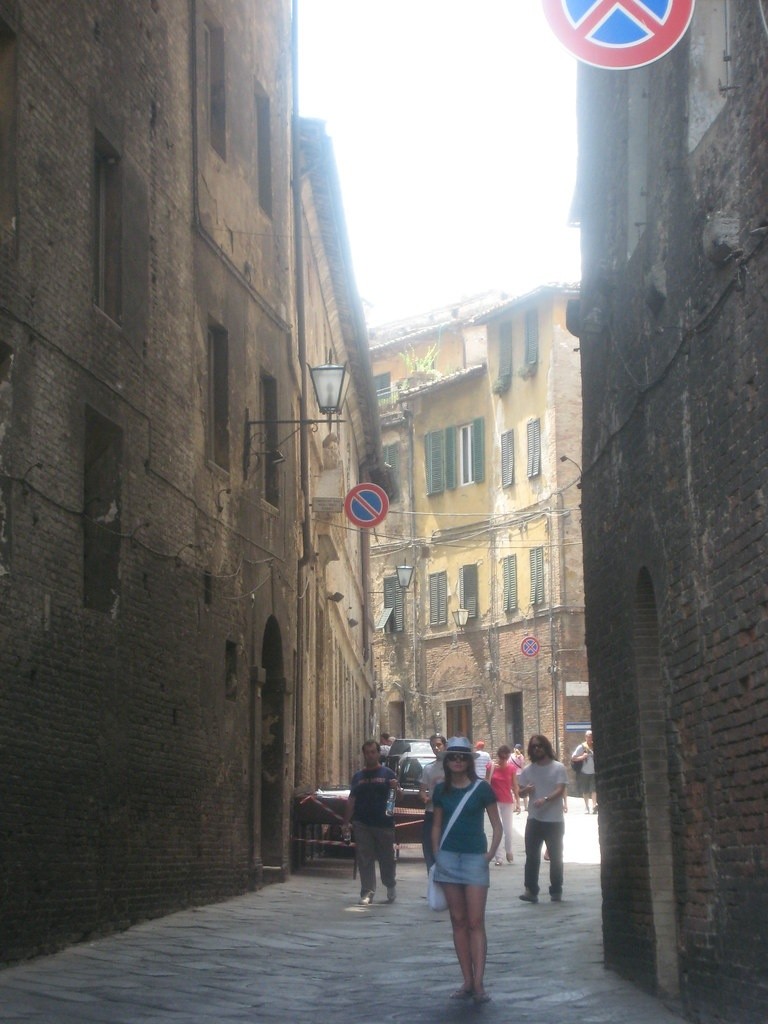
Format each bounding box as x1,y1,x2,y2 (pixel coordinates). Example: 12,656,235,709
544,796,549,803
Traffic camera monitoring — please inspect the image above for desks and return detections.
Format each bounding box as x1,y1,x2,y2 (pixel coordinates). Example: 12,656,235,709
393,807,426,861
294,790,364,880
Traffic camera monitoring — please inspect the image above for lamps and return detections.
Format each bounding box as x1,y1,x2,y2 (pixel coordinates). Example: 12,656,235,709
366,557,413,593
452,605,469,628
244,348,352,481
347,618,358,627
326,592,344,603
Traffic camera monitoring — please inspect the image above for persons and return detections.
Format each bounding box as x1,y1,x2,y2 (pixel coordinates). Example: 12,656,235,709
341,739,404,906
380,733,398,755
418,733,526,876
432,737,504,1003
516,734,567,903
571,730,598,814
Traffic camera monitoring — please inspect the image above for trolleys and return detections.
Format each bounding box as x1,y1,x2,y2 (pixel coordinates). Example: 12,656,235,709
393,789,430,862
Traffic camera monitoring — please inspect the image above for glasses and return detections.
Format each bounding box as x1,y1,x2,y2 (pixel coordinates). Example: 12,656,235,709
448,754,470,761
530,743,544,749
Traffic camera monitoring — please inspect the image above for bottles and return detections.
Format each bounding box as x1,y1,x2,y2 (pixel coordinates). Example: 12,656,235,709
343,829,350,847
385,788,396,817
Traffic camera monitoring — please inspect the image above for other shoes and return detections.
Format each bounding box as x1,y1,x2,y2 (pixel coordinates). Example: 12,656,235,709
359,891,373,904
495,852,513,866
513,807,529,812
387,886,396,899
550,893,562,900
585,803,598,814
518,888,538,902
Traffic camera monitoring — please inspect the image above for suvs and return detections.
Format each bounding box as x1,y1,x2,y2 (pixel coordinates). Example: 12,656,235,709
387,739,437,789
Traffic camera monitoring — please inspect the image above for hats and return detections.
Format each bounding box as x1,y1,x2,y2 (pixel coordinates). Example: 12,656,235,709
515,744,521,749
477,742,484,750
437,736,480,760
585,731,592,735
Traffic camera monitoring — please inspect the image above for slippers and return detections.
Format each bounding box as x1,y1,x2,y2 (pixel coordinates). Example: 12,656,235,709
449,988,491,1004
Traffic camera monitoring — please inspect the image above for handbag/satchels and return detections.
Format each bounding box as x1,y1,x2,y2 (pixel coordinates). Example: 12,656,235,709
571,759,583,772
428,862,448,912
